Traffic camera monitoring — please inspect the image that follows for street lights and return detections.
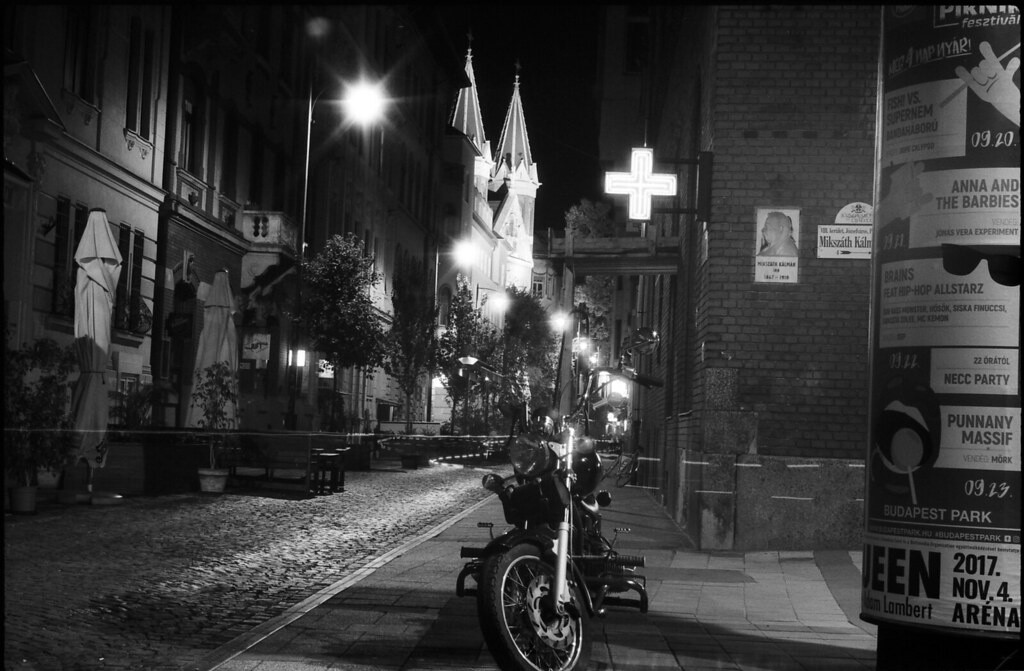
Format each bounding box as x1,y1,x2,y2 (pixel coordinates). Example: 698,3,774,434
425,241,511,423
286,69,395,431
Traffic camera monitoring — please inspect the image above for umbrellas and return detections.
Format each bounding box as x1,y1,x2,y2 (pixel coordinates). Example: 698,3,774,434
182,271,242,432
72,208,125,494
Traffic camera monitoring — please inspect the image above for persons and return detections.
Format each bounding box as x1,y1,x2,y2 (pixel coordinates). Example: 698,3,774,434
756,211,799,257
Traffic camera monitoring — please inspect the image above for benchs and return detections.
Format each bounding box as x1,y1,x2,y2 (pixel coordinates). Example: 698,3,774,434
252,433,353,489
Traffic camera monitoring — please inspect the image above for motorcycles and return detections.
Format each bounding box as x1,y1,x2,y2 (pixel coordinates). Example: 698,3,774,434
454,328,666,671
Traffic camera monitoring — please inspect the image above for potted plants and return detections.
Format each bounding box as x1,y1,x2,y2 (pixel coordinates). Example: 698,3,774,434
1,338,82,514
190,361,237,496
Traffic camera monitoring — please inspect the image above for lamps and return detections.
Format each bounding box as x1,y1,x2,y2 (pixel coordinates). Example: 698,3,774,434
604,147,712,223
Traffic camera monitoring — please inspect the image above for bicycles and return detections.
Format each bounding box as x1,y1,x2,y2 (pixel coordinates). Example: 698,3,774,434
601,439,646,488
